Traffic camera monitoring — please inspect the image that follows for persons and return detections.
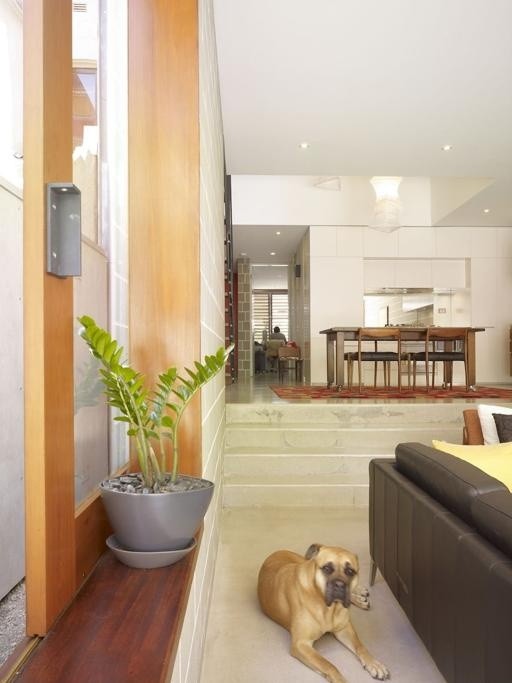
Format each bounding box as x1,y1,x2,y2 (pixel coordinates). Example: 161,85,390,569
269,326,287,344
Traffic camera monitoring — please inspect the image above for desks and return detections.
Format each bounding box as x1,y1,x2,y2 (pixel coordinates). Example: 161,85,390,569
320,327,485,393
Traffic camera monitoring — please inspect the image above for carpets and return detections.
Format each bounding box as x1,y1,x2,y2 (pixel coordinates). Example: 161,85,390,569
271,386,512,399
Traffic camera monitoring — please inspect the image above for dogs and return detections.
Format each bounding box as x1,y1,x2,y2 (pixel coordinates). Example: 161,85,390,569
257,543,389,683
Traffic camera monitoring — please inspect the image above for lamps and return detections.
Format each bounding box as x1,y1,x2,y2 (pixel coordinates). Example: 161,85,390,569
369,176,403,234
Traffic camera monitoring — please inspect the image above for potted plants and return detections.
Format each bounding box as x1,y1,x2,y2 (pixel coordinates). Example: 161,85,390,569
75,315,237,551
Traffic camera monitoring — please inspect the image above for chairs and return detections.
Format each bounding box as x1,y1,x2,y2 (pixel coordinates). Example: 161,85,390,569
344,327,469,394
255,340,303,384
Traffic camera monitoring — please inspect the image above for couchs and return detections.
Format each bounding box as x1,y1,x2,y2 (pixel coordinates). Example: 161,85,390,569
369,443,512,682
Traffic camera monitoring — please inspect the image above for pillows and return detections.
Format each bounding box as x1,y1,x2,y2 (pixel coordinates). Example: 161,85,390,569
396,404,512,555
